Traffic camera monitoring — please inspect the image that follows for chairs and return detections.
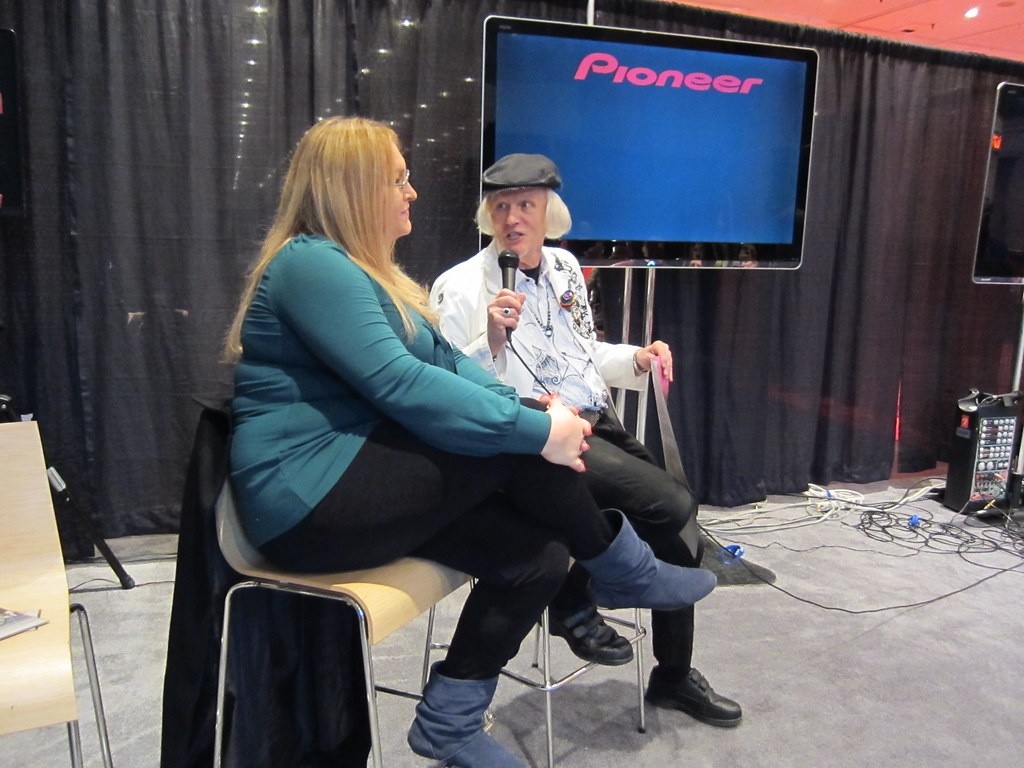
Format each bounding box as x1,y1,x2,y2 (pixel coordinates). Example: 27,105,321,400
1,422,114,768
195,386,647,768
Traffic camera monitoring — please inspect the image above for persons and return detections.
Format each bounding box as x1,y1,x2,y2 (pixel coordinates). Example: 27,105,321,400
226,115,719,768
426,151,743,729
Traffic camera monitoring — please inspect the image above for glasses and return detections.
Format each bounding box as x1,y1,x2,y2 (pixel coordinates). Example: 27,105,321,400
394,169,410,189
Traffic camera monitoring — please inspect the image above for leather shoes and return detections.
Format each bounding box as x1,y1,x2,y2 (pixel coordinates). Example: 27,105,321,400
537,603,633,665
645,666,742,725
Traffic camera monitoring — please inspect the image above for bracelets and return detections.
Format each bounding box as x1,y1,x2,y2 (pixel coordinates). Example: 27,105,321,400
634,353,648,375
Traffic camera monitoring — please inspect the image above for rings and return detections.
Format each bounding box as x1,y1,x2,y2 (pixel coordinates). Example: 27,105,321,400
504,307,510,315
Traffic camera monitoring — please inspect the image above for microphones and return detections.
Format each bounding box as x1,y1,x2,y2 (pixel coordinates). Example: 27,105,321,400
498,248,520,342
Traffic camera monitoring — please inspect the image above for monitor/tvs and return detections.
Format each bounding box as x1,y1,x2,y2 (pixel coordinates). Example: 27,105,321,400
971,82,1024,285
480,16,820,270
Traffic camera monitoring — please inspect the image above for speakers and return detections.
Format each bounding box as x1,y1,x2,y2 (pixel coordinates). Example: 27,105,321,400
0,30,28,222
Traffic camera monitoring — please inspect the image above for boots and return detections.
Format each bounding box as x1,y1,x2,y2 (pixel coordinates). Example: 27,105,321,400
407,660,526,768
569,508,717,610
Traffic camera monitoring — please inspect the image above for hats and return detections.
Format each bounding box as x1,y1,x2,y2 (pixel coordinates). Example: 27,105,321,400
482,153,562,189
